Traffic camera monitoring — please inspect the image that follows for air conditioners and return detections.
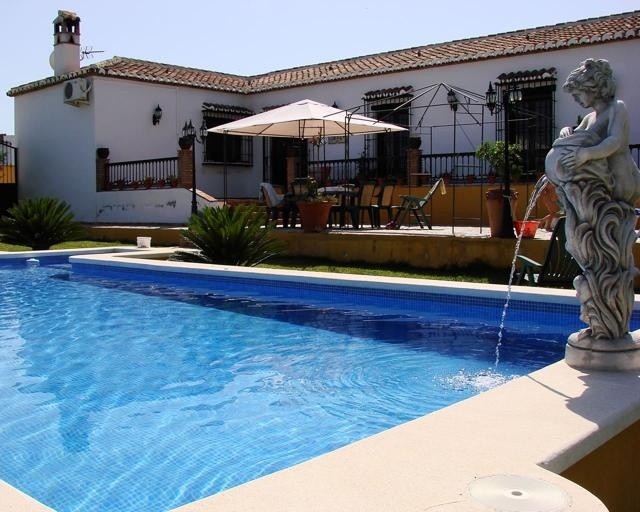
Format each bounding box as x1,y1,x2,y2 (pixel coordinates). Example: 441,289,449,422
63,78,88,103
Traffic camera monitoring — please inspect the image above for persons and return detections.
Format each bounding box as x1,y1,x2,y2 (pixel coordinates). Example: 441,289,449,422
559,58,640,339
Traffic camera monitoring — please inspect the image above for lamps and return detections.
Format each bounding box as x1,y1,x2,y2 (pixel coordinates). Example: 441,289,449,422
153,104,163,125
447,88,458,112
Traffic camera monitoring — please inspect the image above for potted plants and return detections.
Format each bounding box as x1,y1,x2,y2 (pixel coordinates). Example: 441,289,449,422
475,141,523,238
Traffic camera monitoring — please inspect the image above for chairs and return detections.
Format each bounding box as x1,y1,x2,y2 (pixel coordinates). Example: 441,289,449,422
259,176,444,231
514,216,580,291
